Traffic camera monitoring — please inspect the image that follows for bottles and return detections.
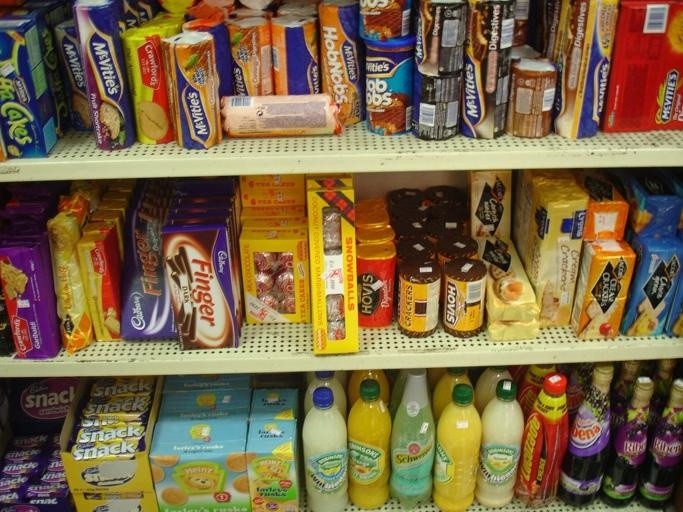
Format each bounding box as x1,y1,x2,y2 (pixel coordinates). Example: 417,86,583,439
301,359,682,512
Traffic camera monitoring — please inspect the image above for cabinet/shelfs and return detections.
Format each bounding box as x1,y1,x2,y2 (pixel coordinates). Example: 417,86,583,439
0,118,683,512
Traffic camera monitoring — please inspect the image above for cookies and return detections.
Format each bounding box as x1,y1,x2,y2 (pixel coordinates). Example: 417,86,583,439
151,453,285,505
